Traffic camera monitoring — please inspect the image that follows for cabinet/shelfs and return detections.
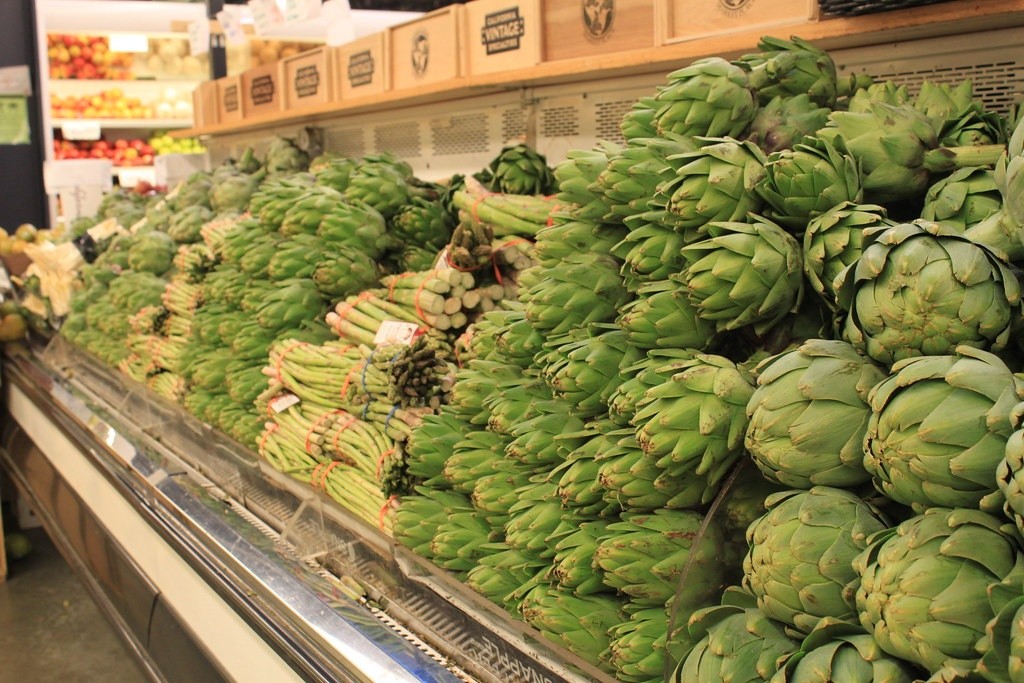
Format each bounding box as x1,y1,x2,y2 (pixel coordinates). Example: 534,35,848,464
35,0,210,194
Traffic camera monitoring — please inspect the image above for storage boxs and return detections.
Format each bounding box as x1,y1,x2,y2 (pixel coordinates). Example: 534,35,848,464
192,0,819,135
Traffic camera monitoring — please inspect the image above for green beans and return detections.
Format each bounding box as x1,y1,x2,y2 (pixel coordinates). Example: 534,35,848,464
251,335,456,541
115,209,249,406
